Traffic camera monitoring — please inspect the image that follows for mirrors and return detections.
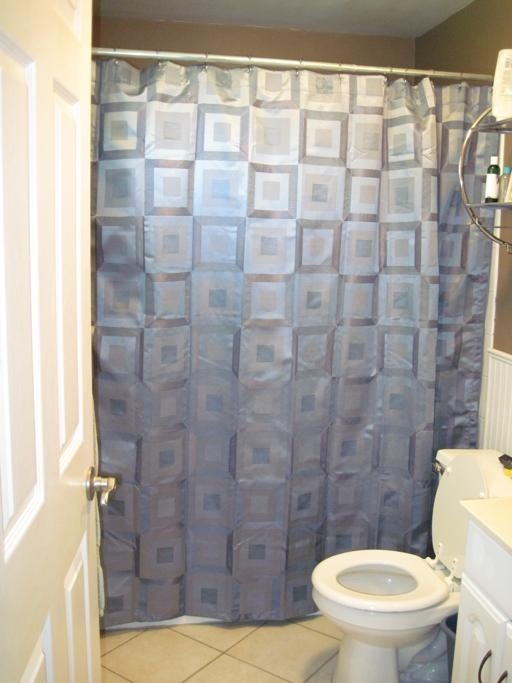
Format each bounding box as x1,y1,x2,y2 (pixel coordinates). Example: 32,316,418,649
484,125,511,361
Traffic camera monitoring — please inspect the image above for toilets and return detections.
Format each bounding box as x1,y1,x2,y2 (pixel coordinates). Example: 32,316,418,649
311,448,512,683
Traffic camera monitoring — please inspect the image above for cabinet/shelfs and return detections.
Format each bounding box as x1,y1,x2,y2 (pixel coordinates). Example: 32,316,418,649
463,116,512,208
452,522,510,682
